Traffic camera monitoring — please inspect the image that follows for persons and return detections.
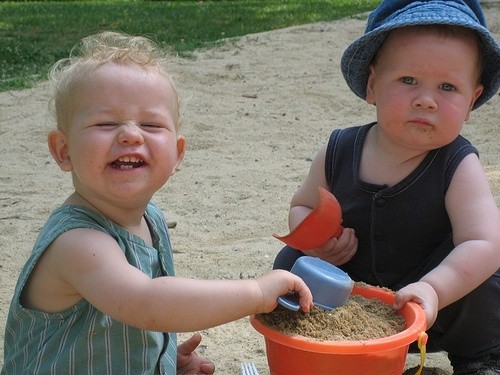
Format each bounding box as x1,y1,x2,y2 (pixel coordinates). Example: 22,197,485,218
272,0,500,375
0,30,315,375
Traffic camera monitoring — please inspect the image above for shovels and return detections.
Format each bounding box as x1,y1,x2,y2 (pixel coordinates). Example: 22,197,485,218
270,186,355,254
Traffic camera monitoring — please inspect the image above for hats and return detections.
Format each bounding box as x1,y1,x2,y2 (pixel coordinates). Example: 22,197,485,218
341,0,499,110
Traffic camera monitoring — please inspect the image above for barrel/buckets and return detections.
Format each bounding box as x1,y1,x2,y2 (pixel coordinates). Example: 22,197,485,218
251,282,428,375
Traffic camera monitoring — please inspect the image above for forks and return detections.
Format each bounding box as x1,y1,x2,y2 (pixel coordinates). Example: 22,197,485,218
241,362,259,375
